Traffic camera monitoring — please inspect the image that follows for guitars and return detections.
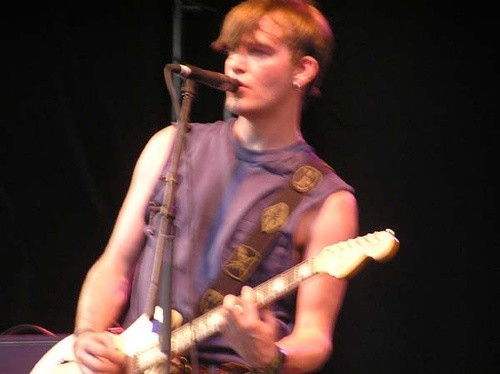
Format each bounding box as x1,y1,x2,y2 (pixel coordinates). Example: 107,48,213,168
29,229,400,374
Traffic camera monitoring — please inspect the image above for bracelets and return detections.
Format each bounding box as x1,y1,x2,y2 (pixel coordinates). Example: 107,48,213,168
265,345,287,374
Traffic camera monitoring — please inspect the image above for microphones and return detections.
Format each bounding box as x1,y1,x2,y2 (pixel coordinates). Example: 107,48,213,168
167,63,238,93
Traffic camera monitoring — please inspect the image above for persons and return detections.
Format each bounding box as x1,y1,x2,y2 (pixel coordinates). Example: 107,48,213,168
73,0,357,374
